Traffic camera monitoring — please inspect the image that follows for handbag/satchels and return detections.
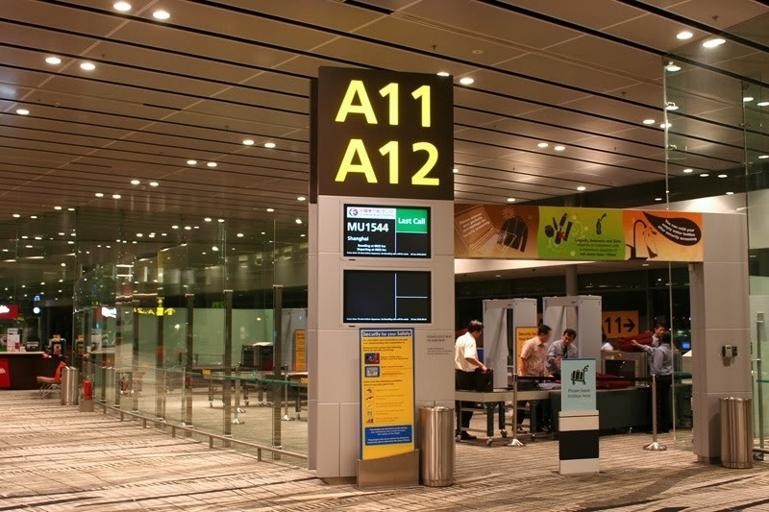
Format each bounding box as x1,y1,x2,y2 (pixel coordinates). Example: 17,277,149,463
474,366,494,392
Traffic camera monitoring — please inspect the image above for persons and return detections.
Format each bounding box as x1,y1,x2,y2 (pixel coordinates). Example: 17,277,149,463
601,332,614,350
630,330,672,432
650,324,665,347
516,326,553,431
454,320,488,440
543,329,578,439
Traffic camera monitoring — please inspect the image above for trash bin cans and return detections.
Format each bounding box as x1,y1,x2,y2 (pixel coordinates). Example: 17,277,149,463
419,406,455,487
61,366,78,405
719,395,754,469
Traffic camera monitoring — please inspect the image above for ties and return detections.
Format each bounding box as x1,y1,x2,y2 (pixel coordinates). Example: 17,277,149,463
562,345,568,358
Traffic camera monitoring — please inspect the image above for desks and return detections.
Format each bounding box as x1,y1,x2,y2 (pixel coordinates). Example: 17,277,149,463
457,383,652,447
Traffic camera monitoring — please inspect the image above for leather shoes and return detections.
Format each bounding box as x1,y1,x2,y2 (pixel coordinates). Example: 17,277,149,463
455,430,477,441
